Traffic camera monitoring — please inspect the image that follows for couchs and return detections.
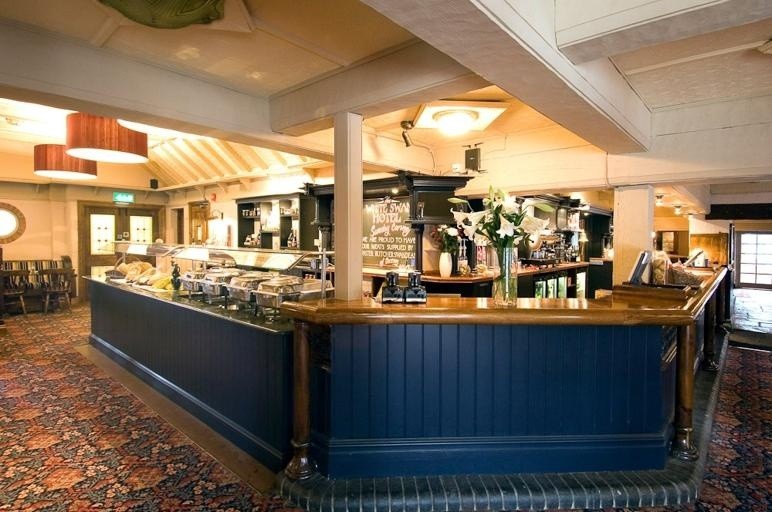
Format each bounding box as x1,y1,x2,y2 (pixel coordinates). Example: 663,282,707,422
0,256,77,298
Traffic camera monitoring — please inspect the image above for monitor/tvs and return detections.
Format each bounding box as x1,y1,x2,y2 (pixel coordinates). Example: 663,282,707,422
415,191,464,219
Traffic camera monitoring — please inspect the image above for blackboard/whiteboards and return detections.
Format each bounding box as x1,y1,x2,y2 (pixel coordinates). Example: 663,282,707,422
363,198,419,278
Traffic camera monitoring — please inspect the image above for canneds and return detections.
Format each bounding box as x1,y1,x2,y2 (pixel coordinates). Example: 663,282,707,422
242,210,253,217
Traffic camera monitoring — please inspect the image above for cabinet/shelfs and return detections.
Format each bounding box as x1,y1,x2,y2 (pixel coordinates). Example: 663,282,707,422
234,193,316,251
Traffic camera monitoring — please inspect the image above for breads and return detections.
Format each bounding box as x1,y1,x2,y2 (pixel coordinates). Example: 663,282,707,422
117,261,174,291
653,259,665,283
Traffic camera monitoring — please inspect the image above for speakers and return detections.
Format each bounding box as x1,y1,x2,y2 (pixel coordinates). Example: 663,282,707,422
465,149,480,171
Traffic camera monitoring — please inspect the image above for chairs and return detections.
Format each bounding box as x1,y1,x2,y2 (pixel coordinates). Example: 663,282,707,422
2,270,74,314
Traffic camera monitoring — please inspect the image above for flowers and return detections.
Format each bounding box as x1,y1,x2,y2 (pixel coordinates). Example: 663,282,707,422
448,188,556,304
429,225,461,253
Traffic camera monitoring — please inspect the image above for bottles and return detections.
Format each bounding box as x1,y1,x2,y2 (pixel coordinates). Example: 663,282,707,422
287,232,297,247
245,233,261,248
242,207,260,217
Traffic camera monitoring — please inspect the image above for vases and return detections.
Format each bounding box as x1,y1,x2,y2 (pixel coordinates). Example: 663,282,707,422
439,250,453,279
491,248,519,310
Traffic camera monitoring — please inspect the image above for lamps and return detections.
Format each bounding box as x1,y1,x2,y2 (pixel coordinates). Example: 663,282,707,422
414,101,510,134
33,113,152,180
401,120,412,146
